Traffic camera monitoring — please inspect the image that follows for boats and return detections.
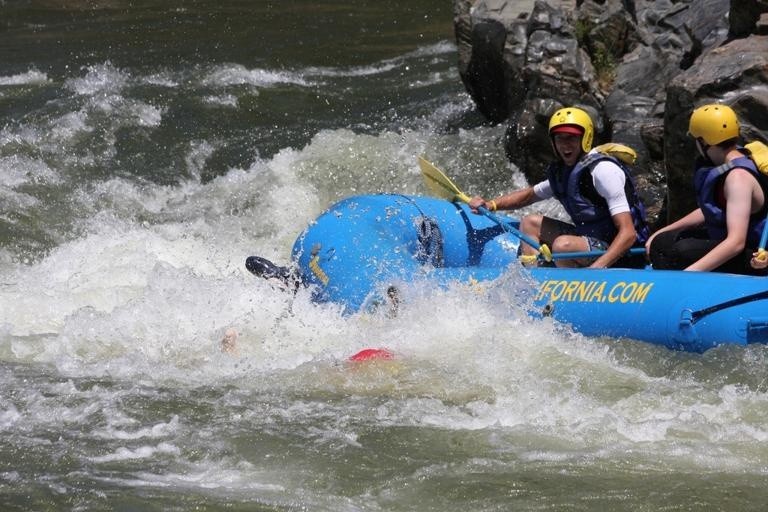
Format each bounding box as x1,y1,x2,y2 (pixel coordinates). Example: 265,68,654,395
291,193,768,355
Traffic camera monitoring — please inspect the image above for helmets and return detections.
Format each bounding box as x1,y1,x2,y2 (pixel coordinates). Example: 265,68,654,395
548,107,594,153
688,104,739,146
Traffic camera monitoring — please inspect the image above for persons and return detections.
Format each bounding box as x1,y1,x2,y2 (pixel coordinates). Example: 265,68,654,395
467,107,651,269
749,247,768,269
644,103,768,272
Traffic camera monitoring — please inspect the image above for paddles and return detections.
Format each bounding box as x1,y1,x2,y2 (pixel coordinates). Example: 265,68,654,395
418,156,552,262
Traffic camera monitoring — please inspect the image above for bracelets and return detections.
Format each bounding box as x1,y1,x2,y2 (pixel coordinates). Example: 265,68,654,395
489,199,497,212
